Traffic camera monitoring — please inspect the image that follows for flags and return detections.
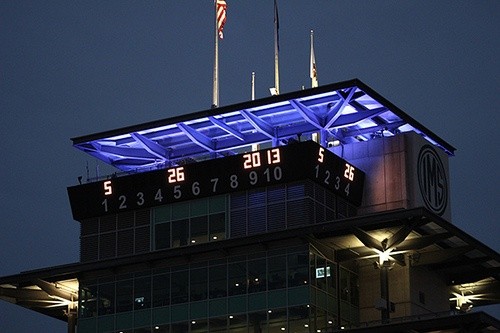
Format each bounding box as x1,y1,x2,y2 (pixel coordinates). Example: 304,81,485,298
216,0,227,40
